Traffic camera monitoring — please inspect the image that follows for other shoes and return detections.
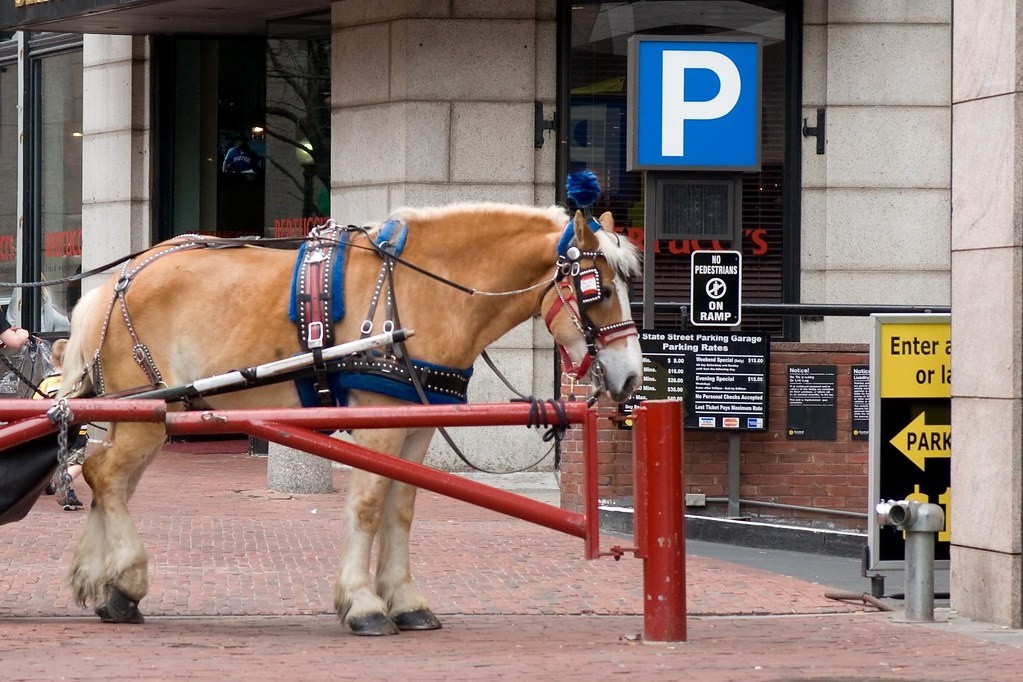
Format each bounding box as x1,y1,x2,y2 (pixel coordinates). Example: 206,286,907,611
63,489,83,510
53,473,68,505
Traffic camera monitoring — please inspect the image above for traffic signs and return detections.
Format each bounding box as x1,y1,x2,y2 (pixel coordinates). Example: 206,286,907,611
868,310,955,571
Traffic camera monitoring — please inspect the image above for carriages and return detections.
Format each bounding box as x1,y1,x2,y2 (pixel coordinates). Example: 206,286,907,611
0,179,648,630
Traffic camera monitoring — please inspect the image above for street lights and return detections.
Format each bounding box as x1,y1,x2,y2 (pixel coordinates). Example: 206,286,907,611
296,135,319,233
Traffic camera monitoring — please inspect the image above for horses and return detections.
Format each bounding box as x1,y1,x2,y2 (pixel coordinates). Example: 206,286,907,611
55,202,646,636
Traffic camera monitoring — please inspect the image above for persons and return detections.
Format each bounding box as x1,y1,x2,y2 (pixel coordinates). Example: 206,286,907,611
220,133,261,174
0,305,29,350
33,339,89,510
